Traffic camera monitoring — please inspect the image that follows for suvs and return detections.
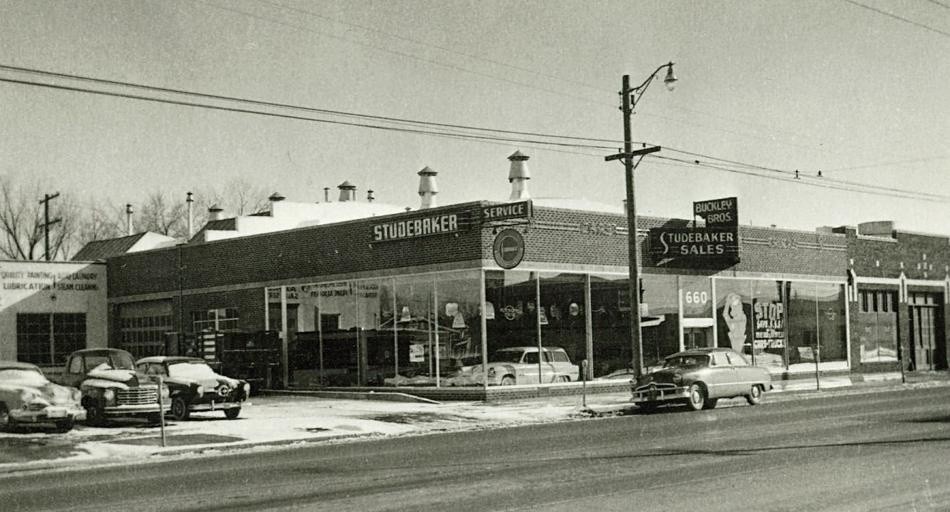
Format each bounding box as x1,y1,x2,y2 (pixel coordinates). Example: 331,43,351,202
57,345,172,429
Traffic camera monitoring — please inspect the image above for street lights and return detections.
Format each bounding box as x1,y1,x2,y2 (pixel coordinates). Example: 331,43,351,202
619,51,680,384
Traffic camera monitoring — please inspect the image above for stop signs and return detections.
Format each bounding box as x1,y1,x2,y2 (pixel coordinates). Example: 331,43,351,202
499,237,520,261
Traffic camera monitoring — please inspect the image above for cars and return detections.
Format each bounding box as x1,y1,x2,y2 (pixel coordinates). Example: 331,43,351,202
0,359,91,436
131,351,254,421
472,342,586,386
623,343,776,414
741,343,784,367
